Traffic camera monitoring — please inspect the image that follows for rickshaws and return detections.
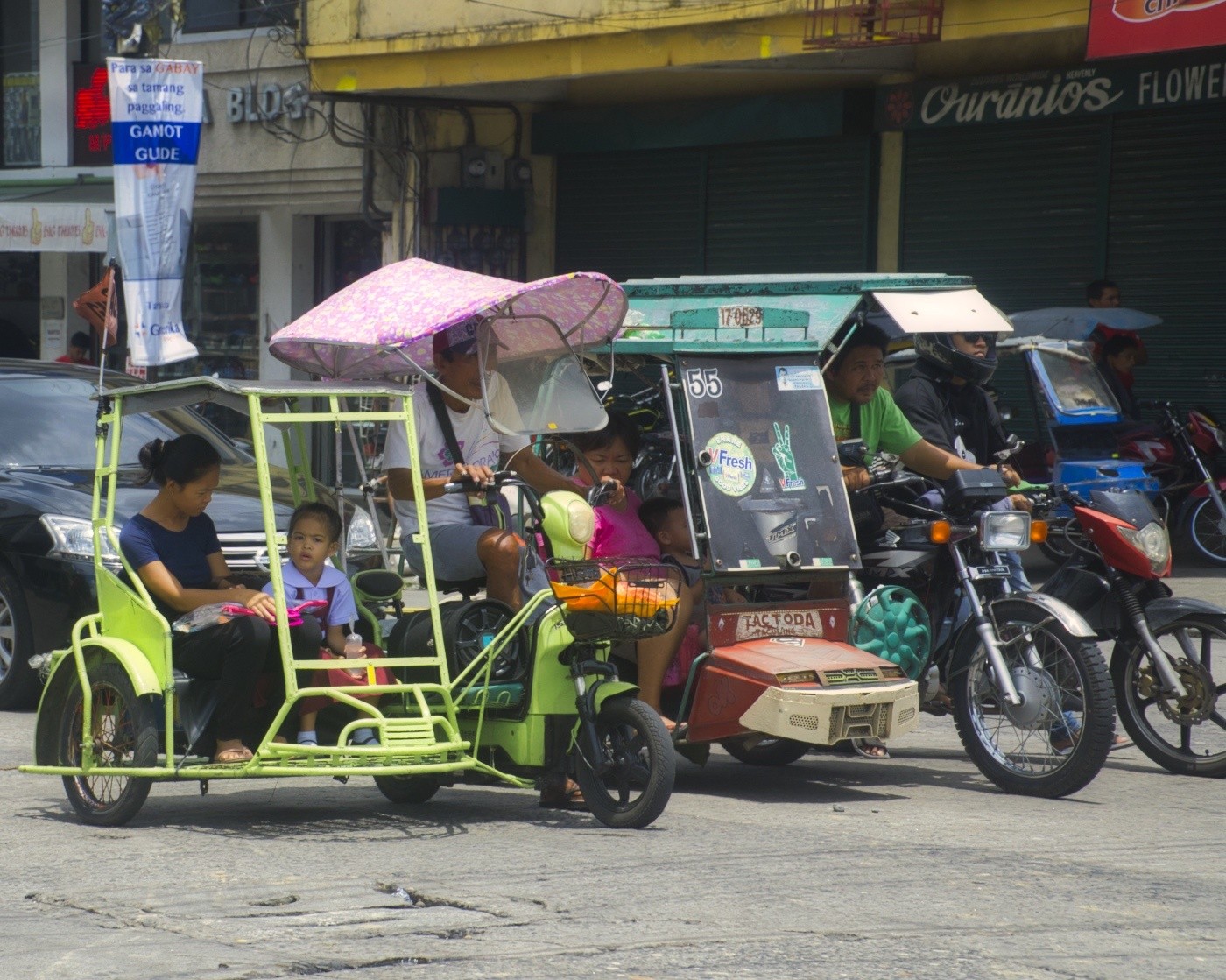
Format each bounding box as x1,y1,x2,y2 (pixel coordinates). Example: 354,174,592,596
12,254,689,830
521,269,1120,800
884,304,1226,781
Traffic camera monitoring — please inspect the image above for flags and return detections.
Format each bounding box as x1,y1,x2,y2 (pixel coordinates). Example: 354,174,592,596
71,265,119,351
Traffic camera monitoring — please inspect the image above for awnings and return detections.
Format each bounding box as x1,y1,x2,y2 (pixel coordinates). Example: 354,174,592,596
0,183,120,253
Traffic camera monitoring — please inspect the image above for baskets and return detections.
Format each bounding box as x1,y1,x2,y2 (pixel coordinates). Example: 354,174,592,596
544,556,681,640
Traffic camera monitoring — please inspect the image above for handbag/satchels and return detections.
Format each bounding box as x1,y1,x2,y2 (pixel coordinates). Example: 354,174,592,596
472,491,513,532
171,599,329,632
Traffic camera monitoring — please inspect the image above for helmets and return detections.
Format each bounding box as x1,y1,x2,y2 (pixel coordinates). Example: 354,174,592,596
912,332,999,386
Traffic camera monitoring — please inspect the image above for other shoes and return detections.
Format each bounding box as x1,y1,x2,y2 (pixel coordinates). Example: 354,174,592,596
1048,710,1136,755
350,737,381,757
297,740,318,747
539,785,588,810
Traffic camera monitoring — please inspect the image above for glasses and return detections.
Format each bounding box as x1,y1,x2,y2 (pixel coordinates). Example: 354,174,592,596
961,332,996,350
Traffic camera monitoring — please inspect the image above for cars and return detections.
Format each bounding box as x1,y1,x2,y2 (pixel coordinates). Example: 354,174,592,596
0,354,382,712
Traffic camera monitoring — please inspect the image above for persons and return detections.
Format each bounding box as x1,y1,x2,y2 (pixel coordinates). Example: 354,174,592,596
1061,280,1177,453
116,433,322,764
260,501,401,758
533,406,750,745
54,330,95,368
893,302,1136,756
819,321,1020,759
379,313,626,812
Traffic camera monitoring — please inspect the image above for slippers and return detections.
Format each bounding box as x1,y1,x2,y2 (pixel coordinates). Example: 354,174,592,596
212,747,254,763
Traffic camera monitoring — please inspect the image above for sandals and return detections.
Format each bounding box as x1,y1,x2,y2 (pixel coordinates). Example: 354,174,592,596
851,736,890,759
661,722,689,741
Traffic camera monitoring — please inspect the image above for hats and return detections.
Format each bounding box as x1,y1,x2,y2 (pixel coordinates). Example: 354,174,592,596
433,313,510,358
